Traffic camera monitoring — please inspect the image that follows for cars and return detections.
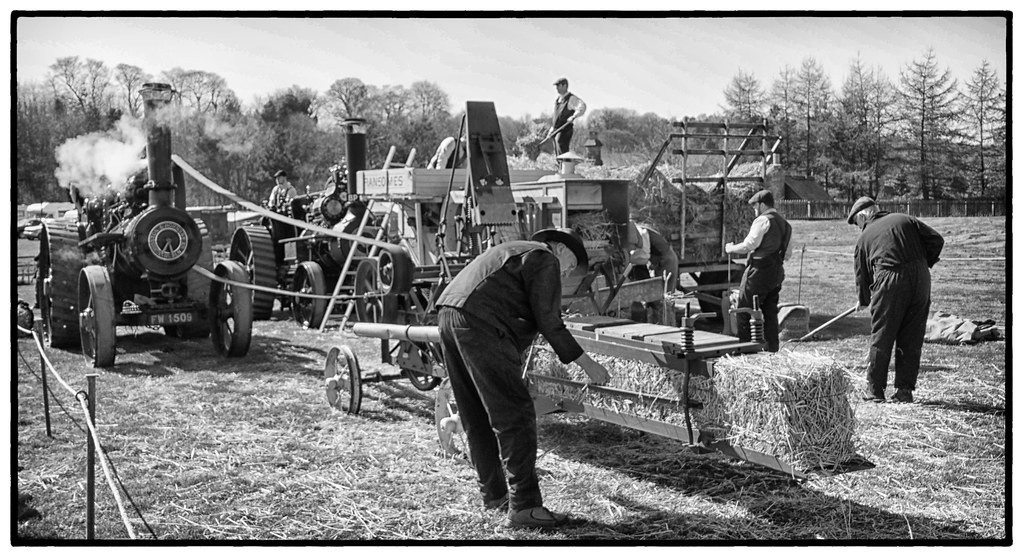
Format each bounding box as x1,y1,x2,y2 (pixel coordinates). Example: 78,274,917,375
16,202,78,240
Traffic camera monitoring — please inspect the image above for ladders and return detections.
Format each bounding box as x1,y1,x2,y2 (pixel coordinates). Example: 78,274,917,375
317,144,417,335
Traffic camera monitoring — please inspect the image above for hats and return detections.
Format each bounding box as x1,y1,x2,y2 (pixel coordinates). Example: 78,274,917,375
532,228,589,276
274,170,287,178
553,78,568,85
847,197,875,225
748,190,772,204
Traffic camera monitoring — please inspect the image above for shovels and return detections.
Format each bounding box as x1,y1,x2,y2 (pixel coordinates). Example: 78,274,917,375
523,119,573,161
779,296,873,353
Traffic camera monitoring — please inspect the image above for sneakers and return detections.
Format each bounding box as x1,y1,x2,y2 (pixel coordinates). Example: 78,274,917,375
862,392,885,402
504,506,568,528
886,387,913,403
483,492,509,510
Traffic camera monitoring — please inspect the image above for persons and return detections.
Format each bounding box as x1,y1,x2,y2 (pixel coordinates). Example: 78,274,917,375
435,227,611,530
628,223,679,327
268,169,298,219
426,133,467,171
725,190,793,353
846,196,945,403
545,77,587,168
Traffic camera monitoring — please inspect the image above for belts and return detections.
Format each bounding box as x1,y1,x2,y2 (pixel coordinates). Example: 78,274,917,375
751,262,783,269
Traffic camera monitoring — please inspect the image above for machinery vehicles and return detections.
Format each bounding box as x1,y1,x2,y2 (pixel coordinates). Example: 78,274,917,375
228,119,398,330
34,82,251,368
